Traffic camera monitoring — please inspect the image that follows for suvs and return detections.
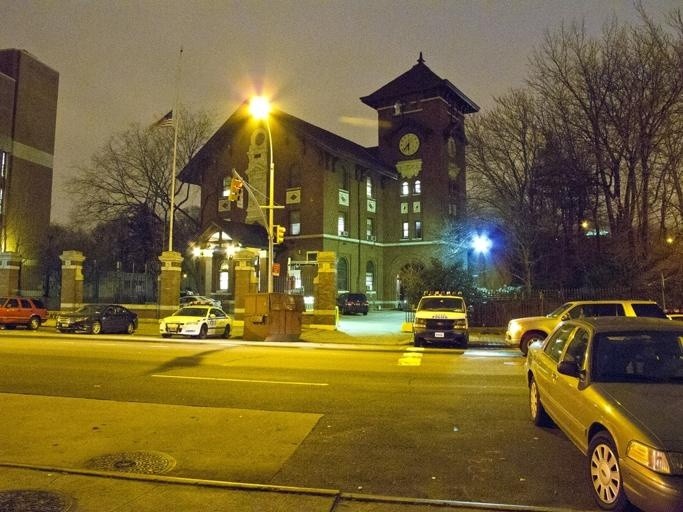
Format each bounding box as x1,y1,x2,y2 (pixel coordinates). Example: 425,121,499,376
412,290,473,348
504,300,671,359
0,295,49,330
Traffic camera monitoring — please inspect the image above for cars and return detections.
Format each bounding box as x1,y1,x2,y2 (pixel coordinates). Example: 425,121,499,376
54,302,138,335
526,315,682,511
158,300,233,338
337,292,368,315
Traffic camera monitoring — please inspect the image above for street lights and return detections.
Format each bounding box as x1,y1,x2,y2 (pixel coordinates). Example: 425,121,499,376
472,234,493,290
246,96,275,293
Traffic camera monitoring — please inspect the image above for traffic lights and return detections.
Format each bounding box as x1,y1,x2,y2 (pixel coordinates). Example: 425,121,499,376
276,224,286,244
228,177,243,202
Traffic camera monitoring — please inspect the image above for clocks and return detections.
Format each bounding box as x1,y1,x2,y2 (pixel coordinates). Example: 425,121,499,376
399,132,420,157
